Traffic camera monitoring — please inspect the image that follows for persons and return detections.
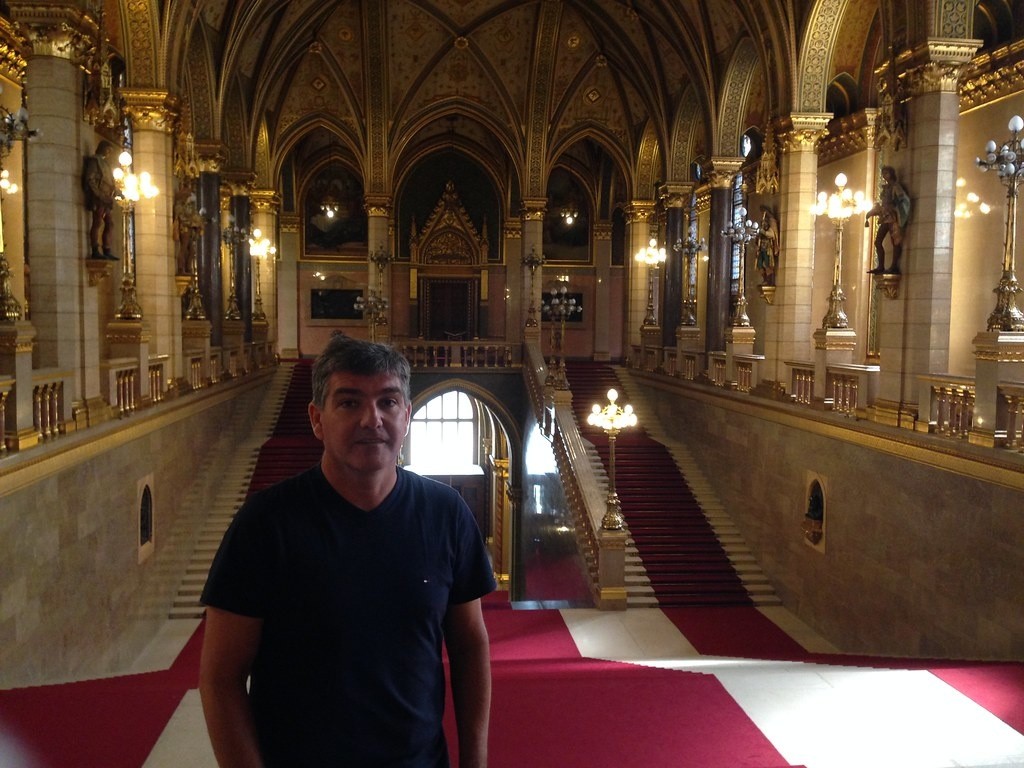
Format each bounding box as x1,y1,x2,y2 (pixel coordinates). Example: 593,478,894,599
864,166,910,274
753,205,780,286
174,177,203,277
196,335,498,768
81,140,125,261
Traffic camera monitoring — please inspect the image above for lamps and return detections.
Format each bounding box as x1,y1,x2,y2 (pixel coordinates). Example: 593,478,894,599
223,214,246,320
543,274,583,390
722,208,759,327
354,286,390,344
370,243,396,297
248,229,276,322
320,130,342,219
536,289,573,385
975,116,1024,332
673,226,705,326
586,389,637,532
809,173,874,327
634,238,667,325
560,204,579,224
520,245,548,327
113,151,159,319
